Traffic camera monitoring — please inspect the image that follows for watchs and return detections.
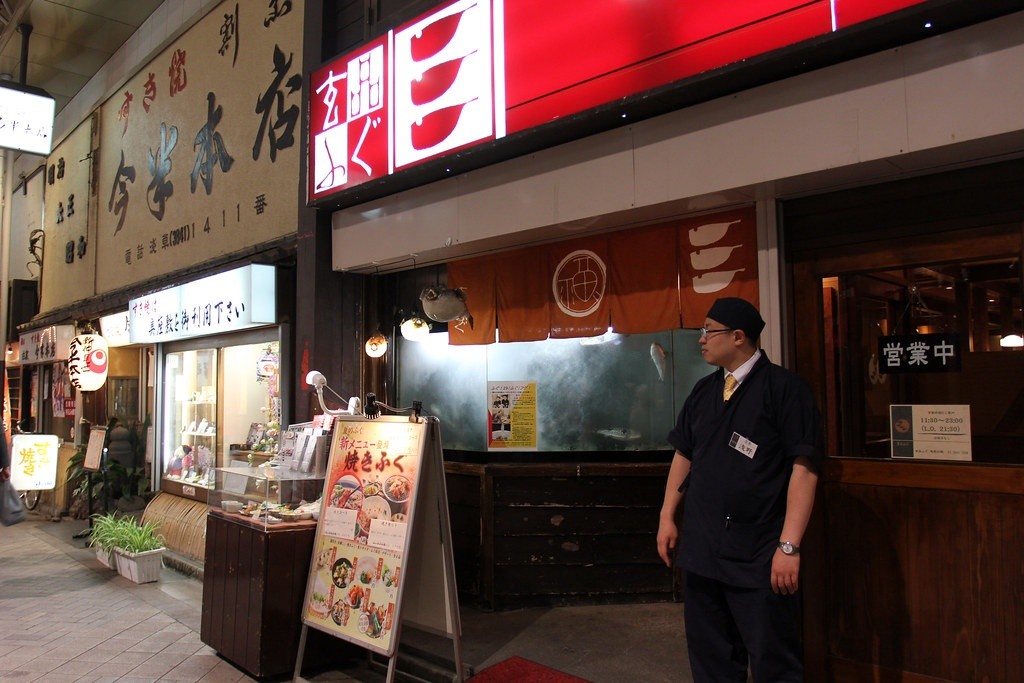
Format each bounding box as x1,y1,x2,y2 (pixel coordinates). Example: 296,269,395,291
777,541,800,555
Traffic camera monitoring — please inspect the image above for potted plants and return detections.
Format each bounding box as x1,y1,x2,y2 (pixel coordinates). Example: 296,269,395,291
103,516,167,584
76,511,136,570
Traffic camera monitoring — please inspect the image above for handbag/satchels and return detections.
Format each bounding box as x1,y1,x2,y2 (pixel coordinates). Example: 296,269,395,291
0,478,26,526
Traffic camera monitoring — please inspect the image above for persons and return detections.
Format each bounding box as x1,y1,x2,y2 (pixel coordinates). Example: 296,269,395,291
0,416,11,483
657,296,825,683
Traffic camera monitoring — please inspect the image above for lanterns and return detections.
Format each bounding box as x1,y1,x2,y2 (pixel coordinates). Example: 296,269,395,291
68,332,108,397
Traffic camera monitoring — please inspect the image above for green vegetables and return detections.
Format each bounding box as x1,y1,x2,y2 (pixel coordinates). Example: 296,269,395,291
382,566,389,580
361,572,366,583
313,593,323,601
386,578,393,586
373,611,380,632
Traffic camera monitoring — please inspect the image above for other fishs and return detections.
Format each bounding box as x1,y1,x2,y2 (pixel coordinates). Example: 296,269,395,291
419,284,474,330
649,340,669,382
596,426,643,442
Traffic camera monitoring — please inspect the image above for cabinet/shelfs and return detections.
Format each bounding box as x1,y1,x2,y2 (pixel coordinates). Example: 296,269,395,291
180,401,216,437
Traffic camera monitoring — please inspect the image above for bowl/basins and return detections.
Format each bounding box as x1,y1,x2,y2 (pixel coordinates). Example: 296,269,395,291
331,600,350,624
382,475,412,515
357,496,392,542
331,558,354,588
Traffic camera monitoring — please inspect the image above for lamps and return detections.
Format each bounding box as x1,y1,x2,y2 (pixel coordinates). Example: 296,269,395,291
365,275,388,358
401,268,432,342
306,371,361,415
364,393,422,423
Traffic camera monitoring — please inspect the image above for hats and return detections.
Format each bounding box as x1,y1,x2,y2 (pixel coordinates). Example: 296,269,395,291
706,297,766,343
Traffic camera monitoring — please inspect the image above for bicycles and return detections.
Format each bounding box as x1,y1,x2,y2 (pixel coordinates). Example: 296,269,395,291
11,418,44,511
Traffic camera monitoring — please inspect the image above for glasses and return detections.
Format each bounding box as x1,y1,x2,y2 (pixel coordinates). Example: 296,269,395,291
700,327,731,337
